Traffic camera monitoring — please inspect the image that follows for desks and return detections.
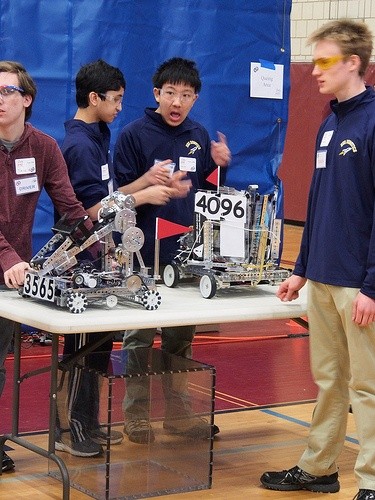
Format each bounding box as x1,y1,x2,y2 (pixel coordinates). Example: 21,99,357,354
0,279,308,500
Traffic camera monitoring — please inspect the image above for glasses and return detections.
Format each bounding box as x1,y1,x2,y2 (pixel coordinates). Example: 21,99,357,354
1,85,26,96
94,91,122,106
158,87,196,104
313,54,359,70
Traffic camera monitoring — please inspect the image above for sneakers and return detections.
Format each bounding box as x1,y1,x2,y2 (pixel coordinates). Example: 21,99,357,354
353,488,375,500
260,464,340,493
0,442,17,472
162,414,219,438
50,425,103,458
86,422,121,443
124,416,153,444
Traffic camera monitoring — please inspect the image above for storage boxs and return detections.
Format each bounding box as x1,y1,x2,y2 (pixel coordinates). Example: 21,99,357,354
47,348,216,500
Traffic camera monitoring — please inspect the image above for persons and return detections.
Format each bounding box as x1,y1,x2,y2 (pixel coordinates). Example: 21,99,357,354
0,59,118,472
260,16,375,500
112,56,232,444
53,58,179,457
